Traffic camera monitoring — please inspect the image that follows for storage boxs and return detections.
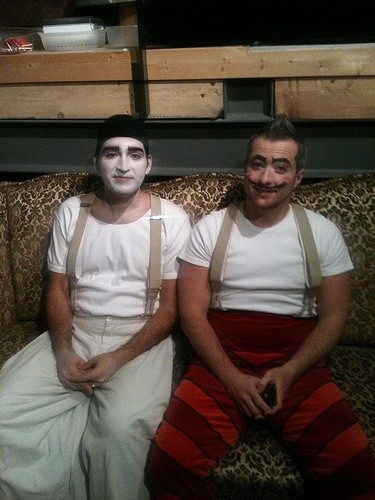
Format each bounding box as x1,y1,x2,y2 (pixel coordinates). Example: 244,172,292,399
37,17,138,52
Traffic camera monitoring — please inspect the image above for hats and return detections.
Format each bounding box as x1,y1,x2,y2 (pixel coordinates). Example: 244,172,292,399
95,114,149,158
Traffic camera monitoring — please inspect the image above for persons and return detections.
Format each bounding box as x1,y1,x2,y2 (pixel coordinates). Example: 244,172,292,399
144,113,375,500
0,114,192,500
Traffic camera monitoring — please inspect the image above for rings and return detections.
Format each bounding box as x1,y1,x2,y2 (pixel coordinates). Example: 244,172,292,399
92,382,95,388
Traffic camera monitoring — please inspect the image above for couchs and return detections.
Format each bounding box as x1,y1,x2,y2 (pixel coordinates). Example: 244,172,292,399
0,173,375,500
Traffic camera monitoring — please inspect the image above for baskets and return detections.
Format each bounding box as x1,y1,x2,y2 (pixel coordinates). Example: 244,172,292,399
37,30,106,52
